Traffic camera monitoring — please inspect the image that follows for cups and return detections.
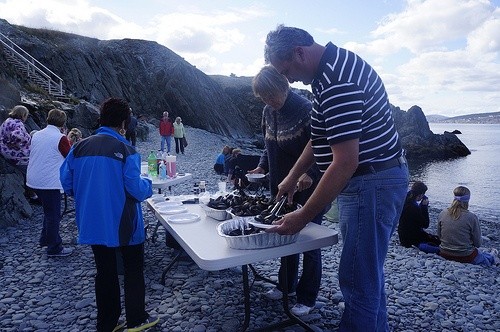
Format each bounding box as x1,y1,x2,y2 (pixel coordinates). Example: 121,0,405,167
219,182,226,193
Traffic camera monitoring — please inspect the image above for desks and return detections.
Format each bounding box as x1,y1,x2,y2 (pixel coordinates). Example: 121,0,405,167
140,173,192,242
147,196,339,332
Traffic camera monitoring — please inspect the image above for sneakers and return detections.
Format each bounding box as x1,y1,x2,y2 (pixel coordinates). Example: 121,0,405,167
291,304,313,315
113,320,125,332
47,247,75,256
128,315,159,331
265,287,296,300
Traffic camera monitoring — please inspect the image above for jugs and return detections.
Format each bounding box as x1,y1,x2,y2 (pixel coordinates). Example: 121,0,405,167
166,155,176,177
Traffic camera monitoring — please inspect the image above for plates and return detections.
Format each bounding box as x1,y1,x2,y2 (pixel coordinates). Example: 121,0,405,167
246,218,281,229
154,201,201,224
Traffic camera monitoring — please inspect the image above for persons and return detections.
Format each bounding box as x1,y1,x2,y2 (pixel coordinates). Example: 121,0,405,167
58,96,161,332
158,111,174,155
262,25,409,332
65,127,84,148
438,186,500,265
398,180,440,249
125,107,151,148
23,109,79,256
213,146,250,190
239,63,334,315
0,105,45,205
172,117,187,155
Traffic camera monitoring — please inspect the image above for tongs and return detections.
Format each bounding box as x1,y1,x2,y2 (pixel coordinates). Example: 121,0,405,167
268,194,287,215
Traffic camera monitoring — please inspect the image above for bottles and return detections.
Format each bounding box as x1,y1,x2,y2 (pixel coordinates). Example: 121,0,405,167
194,183,199,195
199,181,205,193
147,148,168,179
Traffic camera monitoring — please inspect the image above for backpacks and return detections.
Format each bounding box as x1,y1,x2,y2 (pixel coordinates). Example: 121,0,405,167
182,134,187,146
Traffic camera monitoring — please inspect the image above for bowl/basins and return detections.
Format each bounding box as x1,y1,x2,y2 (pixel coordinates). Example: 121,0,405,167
245,173,266,183
